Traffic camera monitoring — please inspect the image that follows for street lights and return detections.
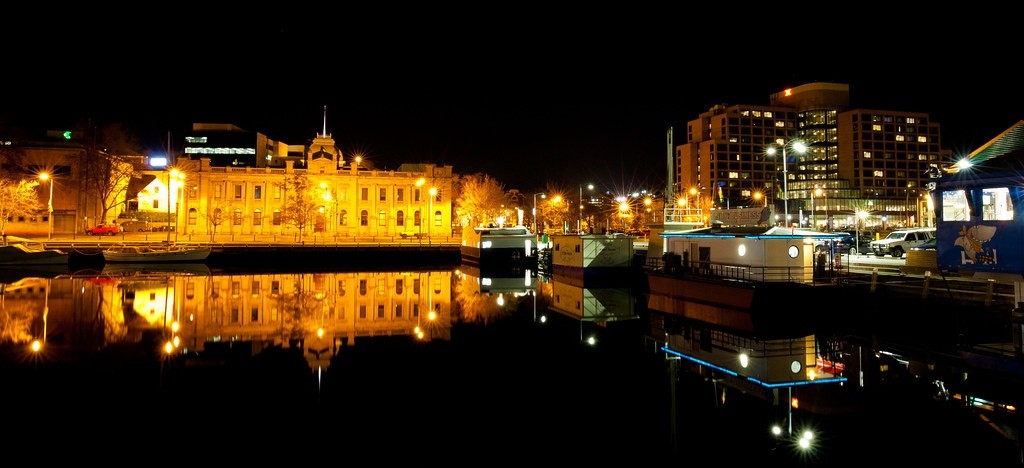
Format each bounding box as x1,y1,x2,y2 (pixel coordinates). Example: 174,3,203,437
427,272,438,321
163,275,184,355
766,139,805,226
416,178,424,246
531,287,547,323
170,168,186,243
578,183,594,233
533,193,546,239
579,319,597,346
26,278,51,355
38,173,55,241
415,270,426,339
429,186,438,247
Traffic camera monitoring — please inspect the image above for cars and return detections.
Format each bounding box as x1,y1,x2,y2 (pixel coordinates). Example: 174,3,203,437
830,234,877,254
85,277,115,288
86,223,119,236
809,234,832,253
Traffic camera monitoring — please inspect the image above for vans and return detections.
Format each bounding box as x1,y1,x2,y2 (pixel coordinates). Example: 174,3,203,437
121,221,150,233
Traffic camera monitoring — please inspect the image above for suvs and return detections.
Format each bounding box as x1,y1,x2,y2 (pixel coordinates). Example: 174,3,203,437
870,230,936,259
